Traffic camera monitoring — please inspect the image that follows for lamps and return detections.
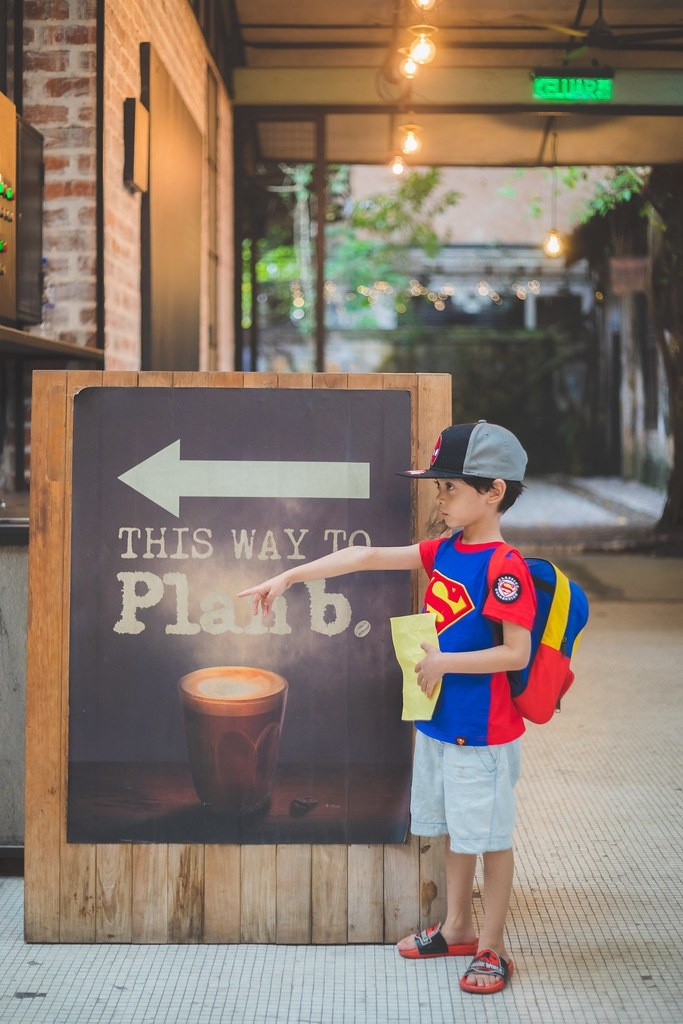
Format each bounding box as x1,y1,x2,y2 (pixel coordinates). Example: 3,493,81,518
382,0,632,257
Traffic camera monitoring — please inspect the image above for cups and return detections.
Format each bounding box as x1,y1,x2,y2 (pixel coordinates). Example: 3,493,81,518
178,666,292,824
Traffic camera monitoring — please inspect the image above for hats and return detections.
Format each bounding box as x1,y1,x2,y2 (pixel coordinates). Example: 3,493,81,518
395,420,528,482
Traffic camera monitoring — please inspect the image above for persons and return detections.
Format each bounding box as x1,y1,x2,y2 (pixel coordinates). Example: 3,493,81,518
235,419,537,996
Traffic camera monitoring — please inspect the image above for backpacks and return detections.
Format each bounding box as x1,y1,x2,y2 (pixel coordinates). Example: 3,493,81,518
488,546,589,724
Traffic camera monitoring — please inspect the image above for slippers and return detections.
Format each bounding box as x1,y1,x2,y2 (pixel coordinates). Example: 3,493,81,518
460,949,513,994
400,921,479,959
